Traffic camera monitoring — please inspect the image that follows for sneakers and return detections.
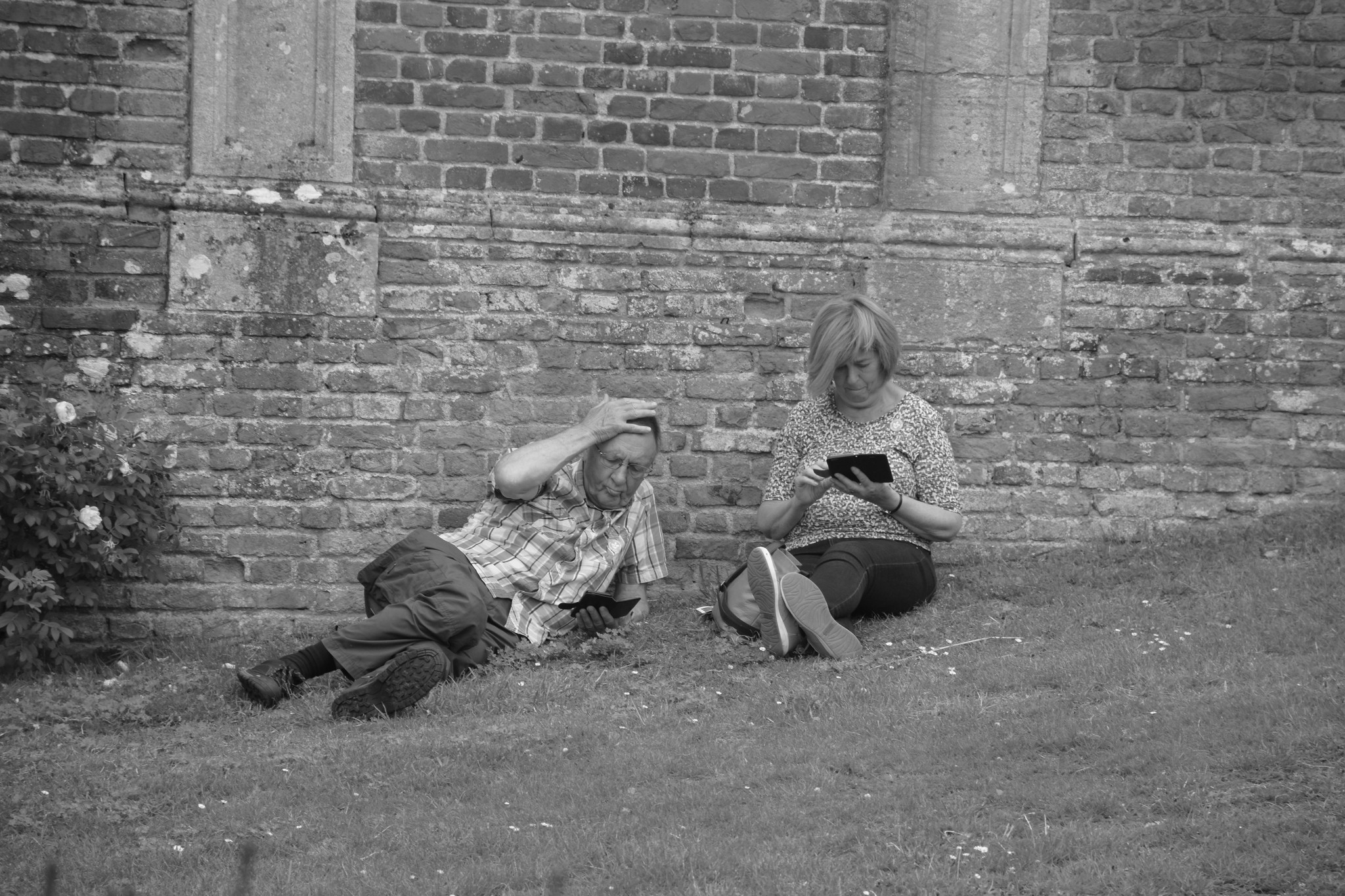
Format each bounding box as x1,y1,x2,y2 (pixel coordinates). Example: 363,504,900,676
331,647,446,726
236,657,311,711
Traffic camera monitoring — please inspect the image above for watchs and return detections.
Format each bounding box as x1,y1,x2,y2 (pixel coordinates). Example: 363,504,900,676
882,492,903,516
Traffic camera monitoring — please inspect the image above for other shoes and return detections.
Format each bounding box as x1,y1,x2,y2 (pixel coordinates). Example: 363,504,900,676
746,547,803,657
780,570,865,663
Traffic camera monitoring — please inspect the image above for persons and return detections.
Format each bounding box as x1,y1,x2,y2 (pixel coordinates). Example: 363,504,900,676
748,294,963,666
235,394,670,720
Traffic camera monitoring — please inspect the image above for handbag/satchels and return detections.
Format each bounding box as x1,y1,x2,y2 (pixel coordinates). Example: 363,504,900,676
710,539,810,644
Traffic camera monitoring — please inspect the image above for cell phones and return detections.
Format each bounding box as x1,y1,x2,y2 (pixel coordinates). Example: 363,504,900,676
826,453,893,483
570,591,641,619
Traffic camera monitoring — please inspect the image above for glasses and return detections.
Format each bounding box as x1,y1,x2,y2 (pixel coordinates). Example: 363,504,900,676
595,442,654,479
